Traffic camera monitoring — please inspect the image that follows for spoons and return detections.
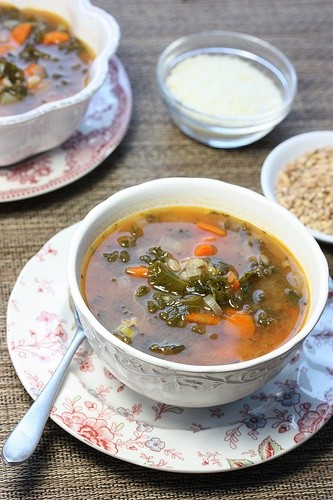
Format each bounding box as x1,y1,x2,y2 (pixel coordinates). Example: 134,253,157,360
2,304,87,466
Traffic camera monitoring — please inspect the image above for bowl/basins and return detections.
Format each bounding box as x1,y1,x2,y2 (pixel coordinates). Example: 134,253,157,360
155,30,298,149
1,0,121,167
68,176,329,409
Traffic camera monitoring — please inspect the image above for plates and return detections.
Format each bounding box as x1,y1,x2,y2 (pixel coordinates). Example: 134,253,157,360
6,221,333,473
1,55,134,202
260,130,333,243
